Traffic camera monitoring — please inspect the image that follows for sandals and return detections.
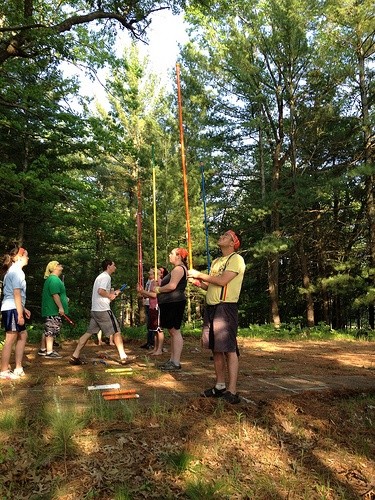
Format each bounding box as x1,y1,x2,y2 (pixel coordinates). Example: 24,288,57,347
68,355,88,365
199,387,226,398
120,355,136,364
223,391,240,404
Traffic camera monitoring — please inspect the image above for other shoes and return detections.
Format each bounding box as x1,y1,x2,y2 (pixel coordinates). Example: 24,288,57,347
140,343,155,349
53,341,59,346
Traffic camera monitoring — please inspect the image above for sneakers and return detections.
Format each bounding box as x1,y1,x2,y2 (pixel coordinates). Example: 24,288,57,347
0,370,20,379
14,367,25,376
38,349,47,356
161,361,181,372
159,359,170,367
45,351,62,358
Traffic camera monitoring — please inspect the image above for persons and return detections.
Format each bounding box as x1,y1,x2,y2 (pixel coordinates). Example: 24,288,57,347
150,248,189,370
0,247,31,380
69,259,136,366
38,261,70,358
136,266,168,356
97,330,116,345
187,229,246,404
